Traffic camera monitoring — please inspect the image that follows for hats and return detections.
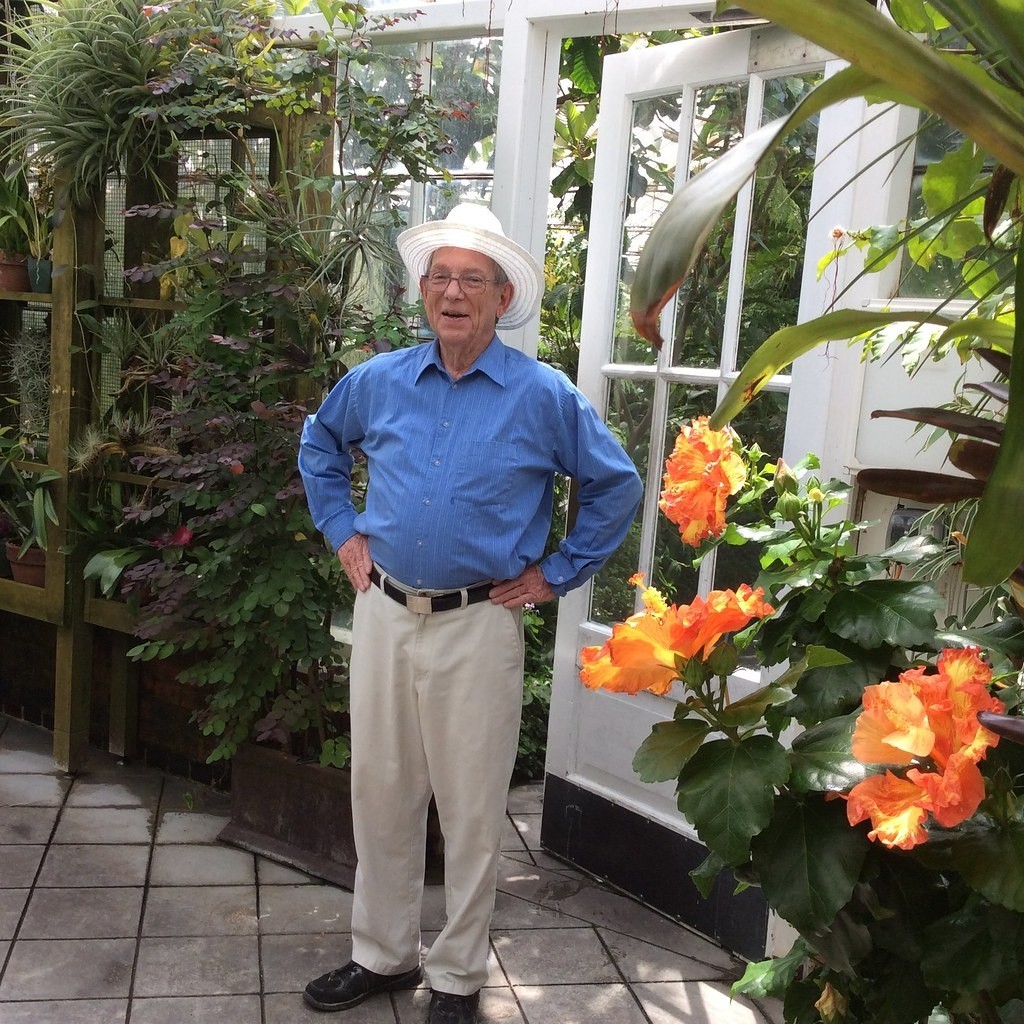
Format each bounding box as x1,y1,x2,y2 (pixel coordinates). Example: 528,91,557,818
396,202,545,330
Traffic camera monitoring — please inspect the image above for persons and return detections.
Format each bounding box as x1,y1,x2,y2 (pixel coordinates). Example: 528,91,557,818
298,202,644,1023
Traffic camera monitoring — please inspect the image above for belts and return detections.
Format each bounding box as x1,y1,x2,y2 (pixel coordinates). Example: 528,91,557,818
367,563,498,612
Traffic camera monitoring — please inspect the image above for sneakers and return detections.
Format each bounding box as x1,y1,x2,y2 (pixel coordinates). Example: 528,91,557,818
301,959,425,1011
424,986,481,1024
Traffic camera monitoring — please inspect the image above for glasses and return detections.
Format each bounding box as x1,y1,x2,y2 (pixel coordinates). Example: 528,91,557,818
421,271,500,296
